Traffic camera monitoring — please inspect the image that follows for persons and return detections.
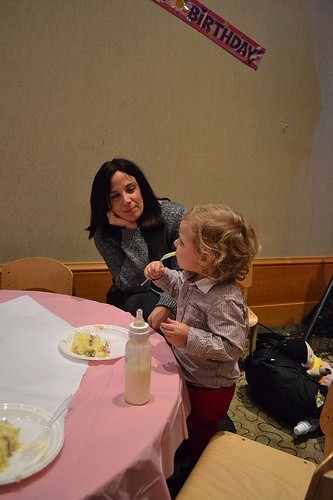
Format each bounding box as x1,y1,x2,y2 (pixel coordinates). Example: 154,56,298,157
145,205,263,479
84,158,187,334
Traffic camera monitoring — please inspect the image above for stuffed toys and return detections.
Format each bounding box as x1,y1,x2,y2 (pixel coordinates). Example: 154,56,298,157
284,338,333,388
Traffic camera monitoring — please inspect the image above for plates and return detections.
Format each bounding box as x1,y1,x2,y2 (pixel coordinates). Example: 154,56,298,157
0,403,64,484
59,324,129,360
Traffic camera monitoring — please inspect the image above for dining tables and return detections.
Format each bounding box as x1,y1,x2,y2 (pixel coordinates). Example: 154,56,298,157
0,291,193,500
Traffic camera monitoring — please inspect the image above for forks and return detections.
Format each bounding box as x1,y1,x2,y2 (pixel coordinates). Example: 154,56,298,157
140,251,176,285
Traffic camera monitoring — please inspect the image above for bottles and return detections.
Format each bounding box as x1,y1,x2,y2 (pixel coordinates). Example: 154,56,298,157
294,417,320,434
124,309,151,405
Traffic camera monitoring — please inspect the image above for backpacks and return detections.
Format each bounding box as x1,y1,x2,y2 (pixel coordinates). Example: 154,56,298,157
243,332,328,425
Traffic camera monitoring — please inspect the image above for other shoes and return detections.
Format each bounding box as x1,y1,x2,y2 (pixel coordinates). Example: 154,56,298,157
173,460,192,480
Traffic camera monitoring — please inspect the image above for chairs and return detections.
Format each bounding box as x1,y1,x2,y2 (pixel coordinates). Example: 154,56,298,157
0,258,74,296
236,263,258,360
174,381,333,499
